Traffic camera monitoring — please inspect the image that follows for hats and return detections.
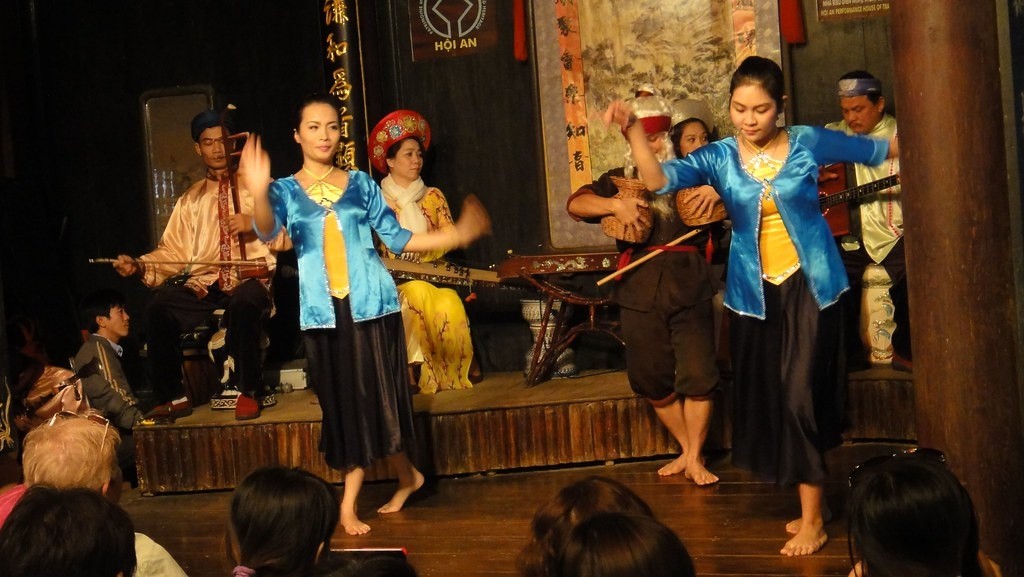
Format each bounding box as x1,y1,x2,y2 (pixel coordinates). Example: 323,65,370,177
368,109,431,174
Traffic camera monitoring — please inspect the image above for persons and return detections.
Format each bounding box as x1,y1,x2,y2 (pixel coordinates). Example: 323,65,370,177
236,93,490,536
604,55,898,557
0,70,1010,577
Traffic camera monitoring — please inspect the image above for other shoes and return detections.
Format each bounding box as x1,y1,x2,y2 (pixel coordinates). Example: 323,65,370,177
468,363,484,384
411,384,421,395
235,393,261,420
150,400,193,417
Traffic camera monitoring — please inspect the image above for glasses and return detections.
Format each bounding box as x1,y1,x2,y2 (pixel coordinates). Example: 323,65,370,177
843,447,947,498
47,410,109,452
